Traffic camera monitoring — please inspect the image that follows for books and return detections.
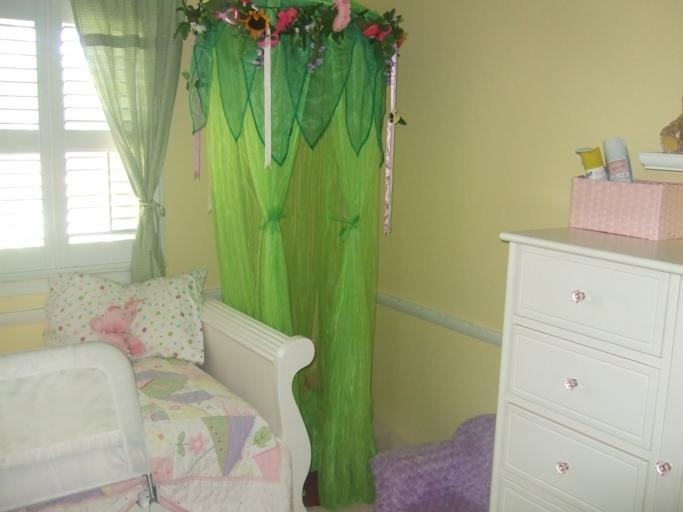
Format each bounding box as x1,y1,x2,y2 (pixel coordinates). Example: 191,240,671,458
566,175,683,245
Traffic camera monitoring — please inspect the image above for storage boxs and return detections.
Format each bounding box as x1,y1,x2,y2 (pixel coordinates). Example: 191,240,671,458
1,298,316,511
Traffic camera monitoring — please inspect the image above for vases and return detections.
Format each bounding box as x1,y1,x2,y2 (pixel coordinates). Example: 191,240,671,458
169,0,406,88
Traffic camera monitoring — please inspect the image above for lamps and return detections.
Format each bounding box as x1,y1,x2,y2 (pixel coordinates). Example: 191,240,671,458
0,341,169,512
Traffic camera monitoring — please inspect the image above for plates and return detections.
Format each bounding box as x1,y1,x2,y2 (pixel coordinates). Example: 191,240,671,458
490,229,682,512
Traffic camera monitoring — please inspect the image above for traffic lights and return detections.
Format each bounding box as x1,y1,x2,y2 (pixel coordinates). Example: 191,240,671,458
43,264,210,368
